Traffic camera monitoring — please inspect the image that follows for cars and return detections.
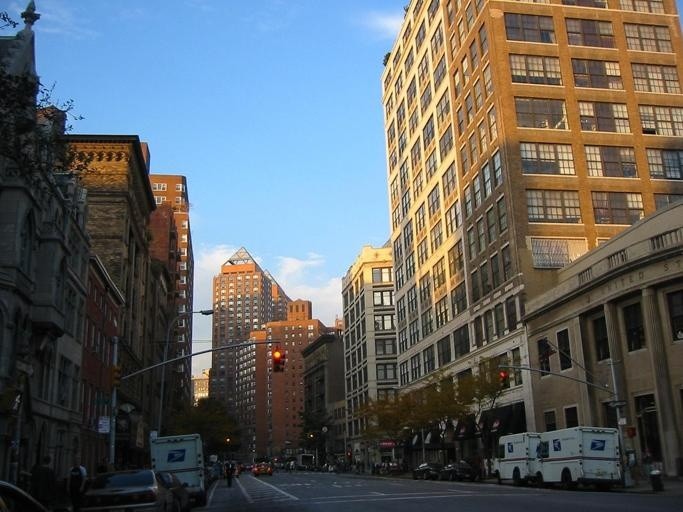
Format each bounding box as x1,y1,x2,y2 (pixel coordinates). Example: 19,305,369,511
76,433,273,511
273,459,476,481
0,479,49,511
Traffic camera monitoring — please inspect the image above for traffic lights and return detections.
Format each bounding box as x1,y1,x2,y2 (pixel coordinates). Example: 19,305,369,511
500,370,507,387
272,350,280,372
280,354,284,372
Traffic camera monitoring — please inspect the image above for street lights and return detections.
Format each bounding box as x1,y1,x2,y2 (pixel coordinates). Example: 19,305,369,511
157,308,215,436
309,432,318,468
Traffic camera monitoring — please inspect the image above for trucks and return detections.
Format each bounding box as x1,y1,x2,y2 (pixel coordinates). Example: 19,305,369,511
493,431,543,486
532,424,622,489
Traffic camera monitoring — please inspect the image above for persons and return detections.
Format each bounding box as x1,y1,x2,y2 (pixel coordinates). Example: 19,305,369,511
64,455,86,511
623,442,641,488
29,453,52,512
223,456,236,488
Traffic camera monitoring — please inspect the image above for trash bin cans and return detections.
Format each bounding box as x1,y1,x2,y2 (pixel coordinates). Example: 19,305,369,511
649,470,664,492
